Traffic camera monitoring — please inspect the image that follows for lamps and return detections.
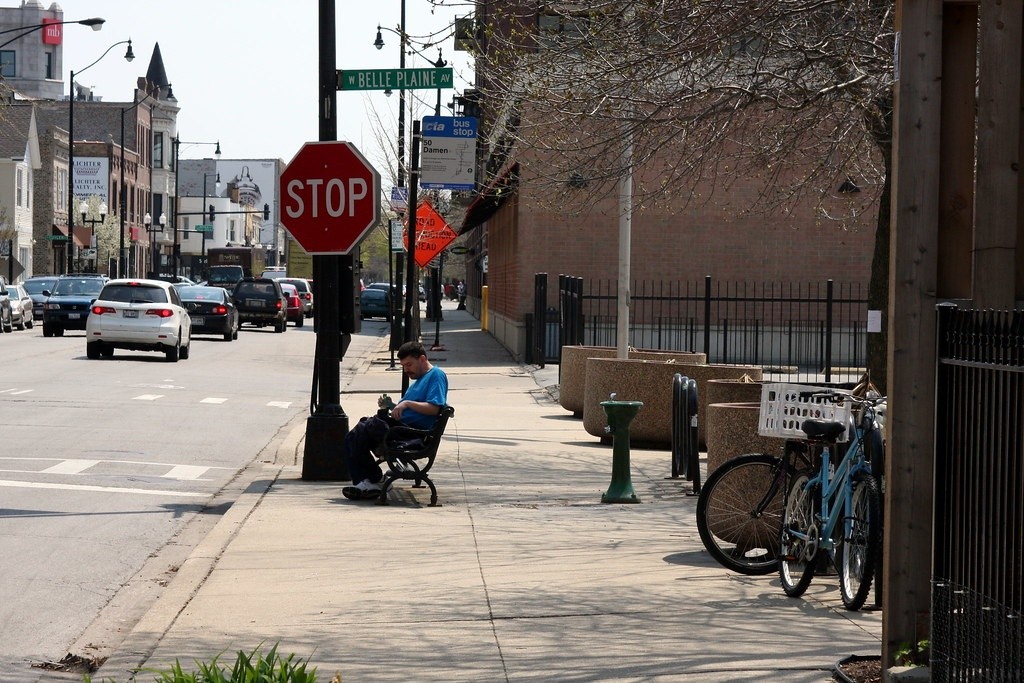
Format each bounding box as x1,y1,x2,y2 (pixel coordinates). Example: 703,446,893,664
567,173,589,188
839,175,861,193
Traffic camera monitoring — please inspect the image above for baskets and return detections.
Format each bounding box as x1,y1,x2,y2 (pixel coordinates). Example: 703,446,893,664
759,383,853,444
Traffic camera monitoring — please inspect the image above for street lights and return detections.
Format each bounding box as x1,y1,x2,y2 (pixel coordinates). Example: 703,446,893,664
143,213,167,273
67,37,137,273
171,132,223,278
118,82,176,279
372,23,450,322
199,171,222,278
79,199,108,273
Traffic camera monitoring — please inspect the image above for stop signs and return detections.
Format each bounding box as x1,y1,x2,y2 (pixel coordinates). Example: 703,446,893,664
278,140,377,256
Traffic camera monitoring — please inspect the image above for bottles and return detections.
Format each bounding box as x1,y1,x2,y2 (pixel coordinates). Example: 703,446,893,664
379,394,388,417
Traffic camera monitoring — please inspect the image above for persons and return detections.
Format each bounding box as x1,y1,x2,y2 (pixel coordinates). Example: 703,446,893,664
457,279,466,311
440,282,456,301
341,342,448,502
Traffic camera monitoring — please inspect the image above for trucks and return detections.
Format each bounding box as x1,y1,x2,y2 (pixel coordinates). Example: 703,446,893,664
206,246,266,295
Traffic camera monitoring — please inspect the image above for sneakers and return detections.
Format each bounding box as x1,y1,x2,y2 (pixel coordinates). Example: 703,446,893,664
376,475,393,491
342,480,381,500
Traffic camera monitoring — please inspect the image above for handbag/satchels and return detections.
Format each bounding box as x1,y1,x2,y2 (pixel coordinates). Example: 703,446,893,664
367,414,425,450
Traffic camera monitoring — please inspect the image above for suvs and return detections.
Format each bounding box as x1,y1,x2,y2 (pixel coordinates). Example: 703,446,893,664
42,273,117,337
230,277,291,333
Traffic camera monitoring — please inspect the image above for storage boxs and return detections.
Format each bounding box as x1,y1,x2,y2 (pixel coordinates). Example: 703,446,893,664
758,384,851,443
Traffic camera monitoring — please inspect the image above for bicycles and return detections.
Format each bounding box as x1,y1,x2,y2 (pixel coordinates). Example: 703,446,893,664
694,367,887,612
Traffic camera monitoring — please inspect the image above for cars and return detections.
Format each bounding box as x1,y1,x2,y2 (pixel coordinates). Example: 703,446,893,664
85,277,197,362
171,283,243,342
0,274,60,334
259,266,426,327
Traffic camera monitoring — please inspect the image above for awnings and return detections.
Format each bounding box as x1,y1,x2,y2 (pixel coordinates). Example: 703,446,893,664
52,223,84,249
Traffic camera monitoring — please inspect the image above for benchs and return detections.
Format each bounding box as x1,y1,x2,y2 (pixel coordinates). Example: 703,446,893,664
374,405,456,506
